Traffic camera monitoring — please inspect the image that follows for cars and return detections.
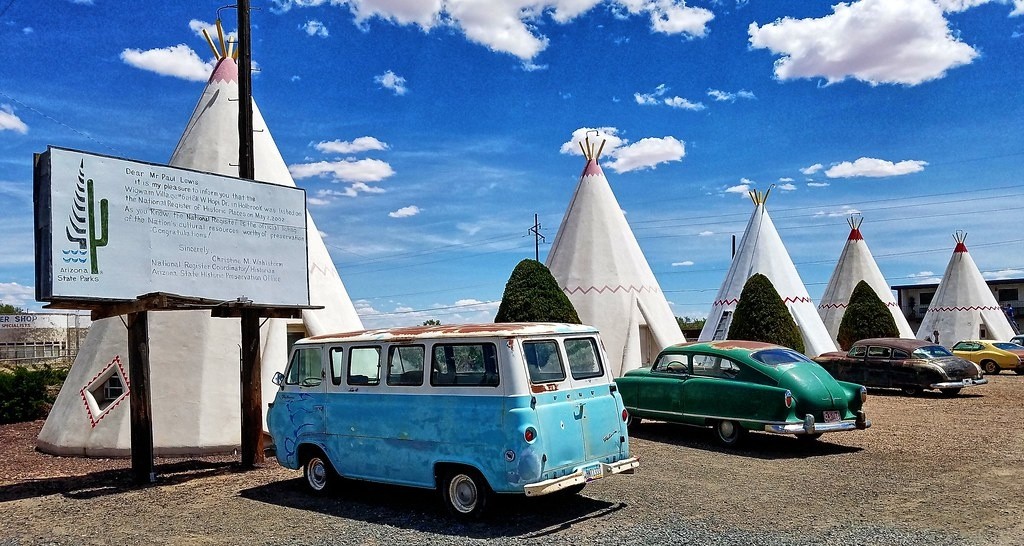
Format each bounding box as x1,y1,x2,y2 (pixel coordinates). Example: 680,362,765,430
611,341,873,448
926,340,1024,376
811,339,988,398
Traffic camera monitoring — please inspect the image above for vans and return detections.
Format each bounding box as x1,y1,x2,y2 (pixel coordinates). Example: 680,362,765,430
266,326,641,524
